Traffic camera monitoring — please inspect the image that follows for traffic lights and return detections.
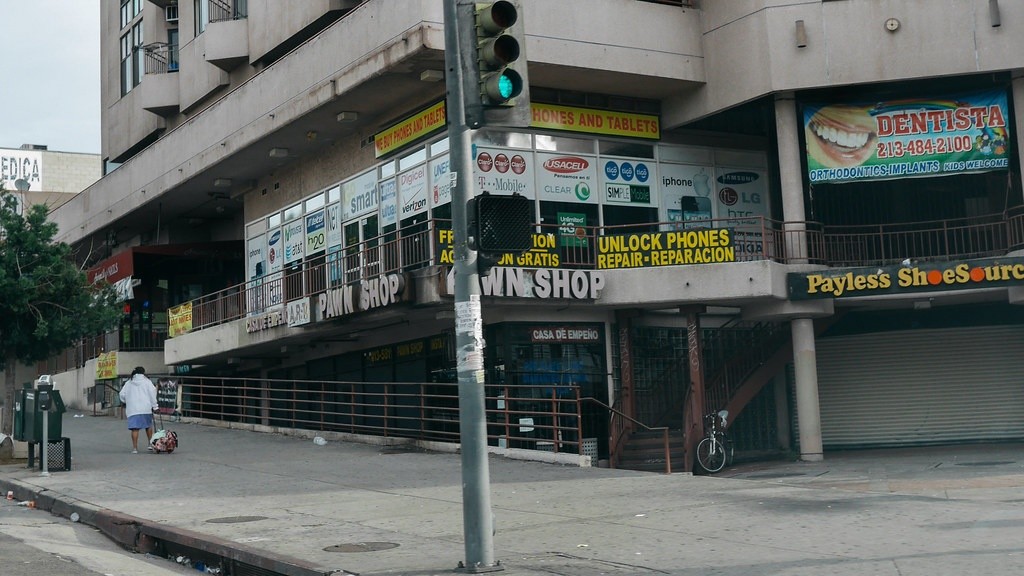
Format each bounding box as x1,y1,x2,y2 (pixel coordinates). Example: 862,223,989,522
475,0,524,108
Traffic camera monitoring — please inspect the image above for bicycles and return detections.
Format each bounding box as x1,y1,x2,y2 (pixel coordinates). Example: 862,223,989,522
696,410,735,473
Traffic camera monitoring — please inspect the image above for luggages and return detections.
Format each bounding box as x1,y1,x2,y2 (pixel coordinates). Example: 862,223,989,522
152,409,179,454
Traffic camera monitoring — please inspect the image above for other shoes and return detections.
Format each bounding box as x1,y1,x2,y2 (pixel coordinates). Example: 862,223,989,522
147,444,153,450
132,448,138,454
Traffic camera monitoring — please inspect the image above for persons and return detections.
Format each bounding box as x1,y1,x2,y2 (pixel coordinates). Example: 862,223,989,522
120,366,158,454
805,107,878,168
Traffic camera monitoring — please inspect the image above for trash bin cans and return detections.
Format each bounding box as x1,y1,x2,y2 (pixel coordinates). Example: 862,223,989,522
536,441,554,451
14,382,66,444
583,438,598,467
